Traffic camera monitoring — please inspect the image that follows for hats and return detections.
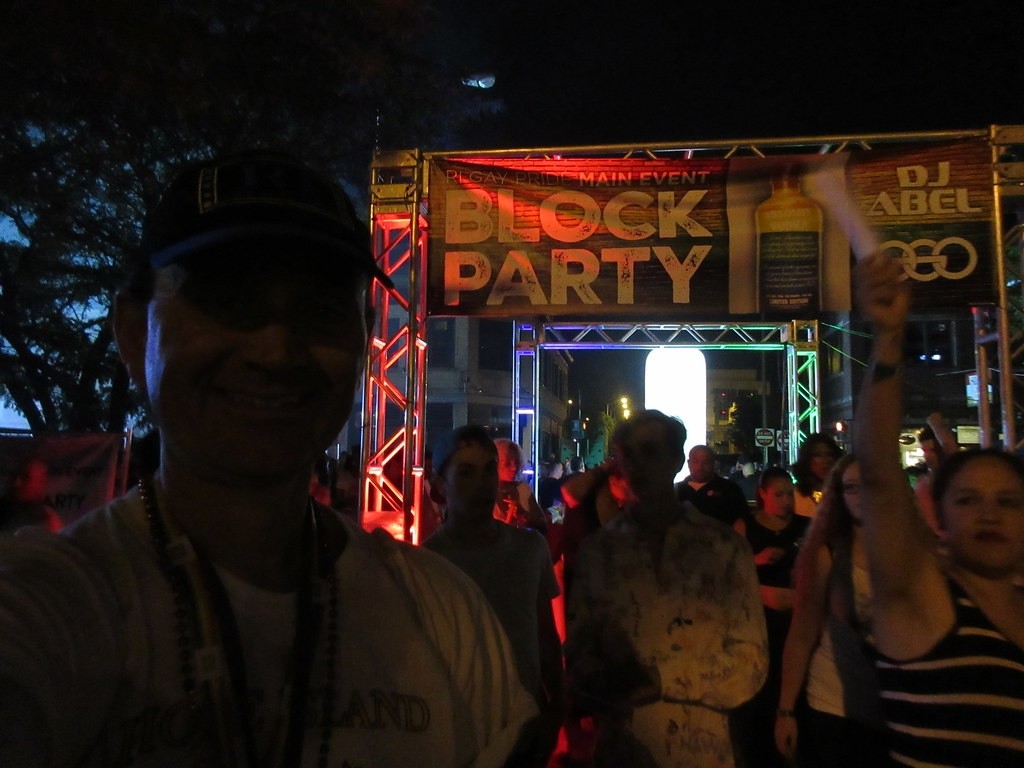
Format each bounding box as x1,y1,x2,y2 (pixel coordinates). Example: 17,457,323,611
129,152,397,287
429,423,500,507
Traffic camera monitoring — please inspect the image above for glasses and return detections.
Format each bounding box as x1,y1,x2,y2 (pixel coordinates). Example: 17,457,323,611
842,482,866,495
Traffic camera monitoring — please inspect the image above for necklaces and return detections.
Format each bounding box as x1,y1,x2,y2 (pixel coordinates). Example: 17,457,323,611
137,474,340,768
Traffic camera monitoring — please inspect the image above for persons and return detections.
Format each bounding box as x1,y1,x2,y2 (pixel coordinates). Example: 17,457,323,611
311,253,1024,768
0,154,535,768
0,459,65,535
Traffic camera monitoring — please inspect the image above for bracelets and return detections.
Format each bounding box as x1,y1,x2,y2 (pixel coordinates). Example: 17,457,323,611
864,360,902,380
777,710,793,716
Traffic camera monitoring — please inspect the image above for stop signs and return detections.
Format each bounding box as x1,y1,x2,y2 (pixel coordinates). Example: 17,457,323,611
776,430,789,452
754,428,774,447
